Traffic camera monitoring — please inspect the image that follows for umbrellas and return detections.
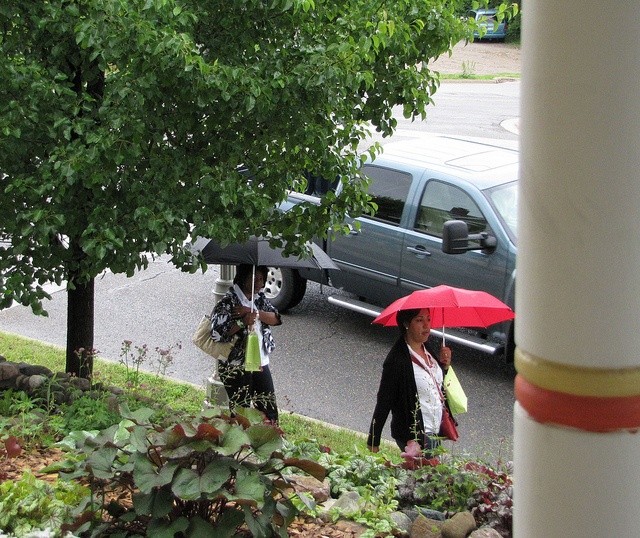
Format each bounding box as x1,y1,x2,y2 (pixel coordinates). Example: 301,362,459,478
166,225,342,331
371,285,515,363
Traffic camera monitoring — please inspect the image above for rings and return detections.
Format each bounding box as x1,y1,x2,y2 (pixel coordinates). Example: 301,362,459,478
238,312,240,315
445,353,448,356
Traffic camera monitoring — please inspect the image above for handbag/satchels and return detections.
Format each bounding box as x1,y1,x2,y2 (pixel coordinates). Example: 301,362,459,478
443,365,467,415
192,296,239,363
245,323,261,371
410,353,459,441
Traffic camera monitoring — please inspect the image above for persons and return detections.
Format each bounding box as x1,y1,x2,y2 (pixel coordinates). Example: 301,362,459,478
210,263,283,429
366,308,459,461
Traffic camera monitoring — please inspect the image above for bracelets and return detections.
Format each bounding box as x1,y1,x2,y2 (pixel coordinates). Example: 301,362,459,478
255,310,260,319
236,318,246,330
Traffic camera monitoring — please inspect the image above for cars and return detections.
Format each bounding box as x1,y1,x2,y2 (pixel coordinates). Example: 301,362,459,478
465,7,507,42
220,129,518,372
